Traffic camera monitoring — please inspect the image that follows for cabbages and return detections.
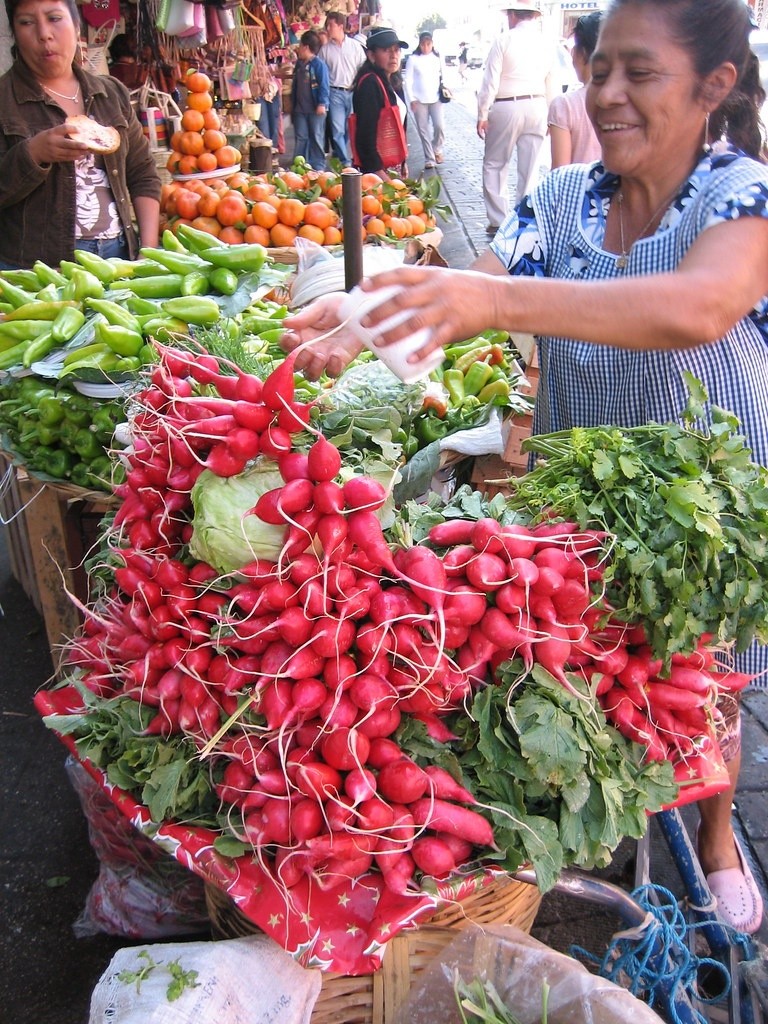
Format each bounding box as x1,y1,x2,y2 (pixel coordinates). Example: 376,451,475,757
189,459,324,576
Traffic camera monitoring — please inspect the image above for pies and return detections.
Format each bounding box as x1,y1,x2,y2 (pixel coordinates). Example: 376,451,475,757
65,114,121,153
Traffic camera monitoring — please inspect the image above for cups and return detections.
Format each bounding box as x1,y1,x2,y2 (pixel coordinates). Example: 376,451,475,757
337,279,445,385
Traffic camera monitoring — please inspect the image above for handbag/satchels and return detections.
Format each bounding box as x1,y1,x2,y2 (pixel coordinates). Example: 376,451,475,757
349,72,407,168
438,76,454,103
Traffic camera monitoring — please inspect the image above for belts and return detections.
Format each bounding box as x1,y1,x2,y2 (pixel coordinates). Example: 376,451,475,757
495,94,545,101
330,86,354,92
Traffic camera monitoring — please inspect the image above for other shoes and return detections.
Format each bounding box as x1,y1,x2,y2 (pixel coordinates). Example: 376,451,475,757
486,225,499,233
344,162,352,167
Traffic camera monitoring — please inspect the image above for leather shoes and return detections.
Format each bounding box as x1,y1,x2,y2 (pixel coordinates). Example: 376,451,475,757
435,153,443,164
425,164,434,169
696,817,764,936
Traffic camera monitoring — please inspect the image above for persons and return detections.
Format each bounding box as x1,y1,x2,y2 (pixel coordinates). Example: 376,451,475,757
0,0,160,271
138,42,181,106
477,0,556,236
289,31,330,171
108,34,150,90
279,0,768,936
317,11,368,167
255,61,286,166
405,32,452,169
457,41,469,82
545,10,604,170
351,27,408,183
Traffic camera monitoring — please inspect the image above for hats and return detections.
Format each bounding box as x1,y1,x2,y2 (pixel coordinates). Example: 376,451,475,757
366,27,409,50
499,0,542,18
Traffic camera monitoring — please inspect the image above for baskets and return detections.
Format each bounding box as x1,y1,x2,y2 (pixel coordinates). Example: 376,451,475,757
200,862,662,1024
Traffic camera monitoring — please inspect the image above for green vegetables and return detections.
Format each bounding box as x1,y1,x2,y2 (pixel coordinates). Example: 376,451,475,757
484,370,768,680
118,950,199,1002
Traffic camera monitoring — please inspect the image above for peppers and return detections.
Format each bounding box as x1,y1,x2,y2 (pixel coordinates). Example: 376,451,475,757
0,223,522,492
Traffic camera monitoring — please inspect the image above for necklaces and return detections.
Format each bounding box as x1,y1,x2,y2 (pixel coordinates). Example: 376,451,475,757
617,184,680,270
40,83,79,104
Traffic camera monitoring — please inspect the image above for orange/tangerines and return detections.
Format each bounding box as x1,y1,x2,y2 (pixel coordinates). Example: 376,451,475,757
167,74,242,175
160,167,436,246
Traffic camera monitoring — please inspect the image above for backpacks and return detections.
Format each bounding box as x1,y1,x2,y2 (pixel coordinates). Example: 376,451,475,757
83,0,380,120
